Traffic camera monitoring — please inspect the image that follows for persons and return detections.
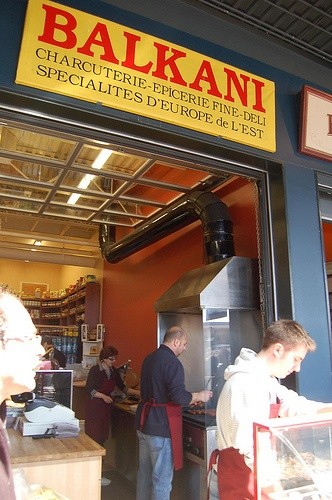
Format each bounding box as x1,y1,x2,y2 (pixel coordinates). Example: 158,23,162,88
0,289,46,500
41,337,67,370
86,345,142,486
207,319,332,500
134,326,213,500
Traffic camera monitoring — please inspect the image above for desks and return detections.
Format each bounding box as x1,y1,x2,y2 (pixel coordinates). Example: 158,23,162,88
6,427,106,500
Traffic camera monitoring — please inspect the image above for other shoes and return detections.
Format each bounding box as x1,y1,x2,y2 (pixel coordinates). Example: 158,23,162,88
101,477,111,486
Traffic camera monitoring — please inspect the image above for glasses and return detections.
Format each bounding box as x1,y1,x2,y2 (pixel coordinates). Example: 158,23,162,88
0,335,42,350
107,357,119,361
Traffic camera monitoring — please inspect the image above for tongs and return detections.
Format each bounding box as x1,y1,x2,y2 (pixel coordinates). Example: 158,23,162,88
205,376,220,391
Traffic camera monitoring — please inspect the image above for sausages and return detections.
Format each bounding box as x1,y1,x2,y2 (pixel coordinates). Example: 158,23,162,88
187,410,216,416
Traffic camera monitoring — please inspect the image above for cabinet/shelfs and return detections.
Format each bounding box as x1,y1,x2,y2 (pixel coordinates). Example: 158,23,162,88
22,281,100,339
